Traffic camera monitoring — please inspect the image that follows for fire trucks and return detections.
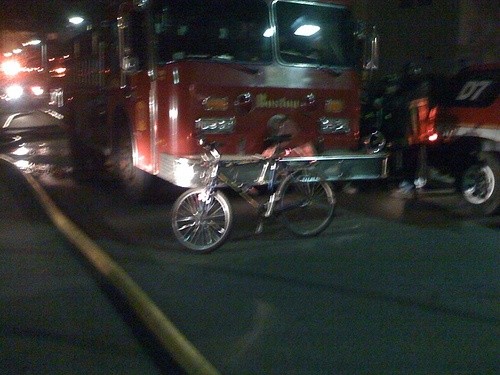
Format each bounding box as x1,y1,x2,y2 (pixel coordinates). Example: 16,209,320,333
66,0,392,206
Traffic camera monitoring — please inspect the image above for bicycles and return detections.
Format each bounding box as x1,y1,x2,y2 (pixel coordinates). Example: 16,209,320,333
170,134,337,251
